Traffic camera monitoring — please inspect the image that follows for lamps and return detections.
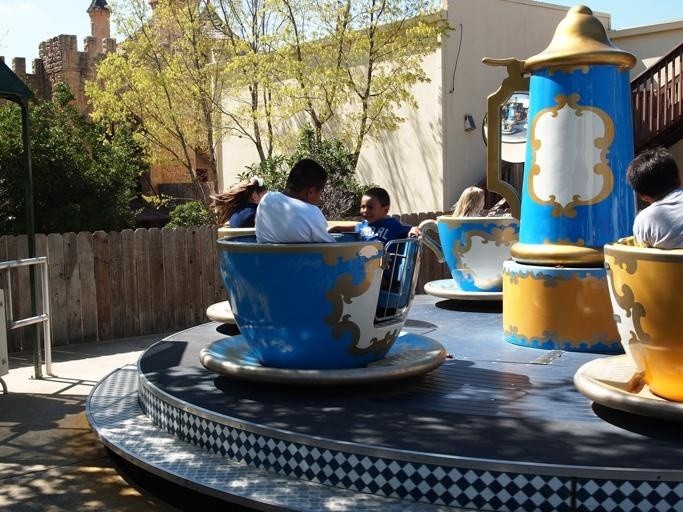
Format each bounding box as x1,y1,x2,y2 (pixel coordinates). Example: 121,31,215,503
465,116,476,131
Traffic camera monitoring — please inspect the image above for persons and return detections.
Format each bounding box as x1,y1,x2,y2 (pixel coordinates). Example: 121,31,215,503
209,176,268,242
621,147,683,250
253,159,356,242
454,185,512,217
327,187,423,289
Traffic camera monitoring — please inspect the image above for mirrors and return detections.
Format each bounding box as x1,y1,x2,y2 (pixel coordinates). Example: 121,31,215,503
482,92,529,164
